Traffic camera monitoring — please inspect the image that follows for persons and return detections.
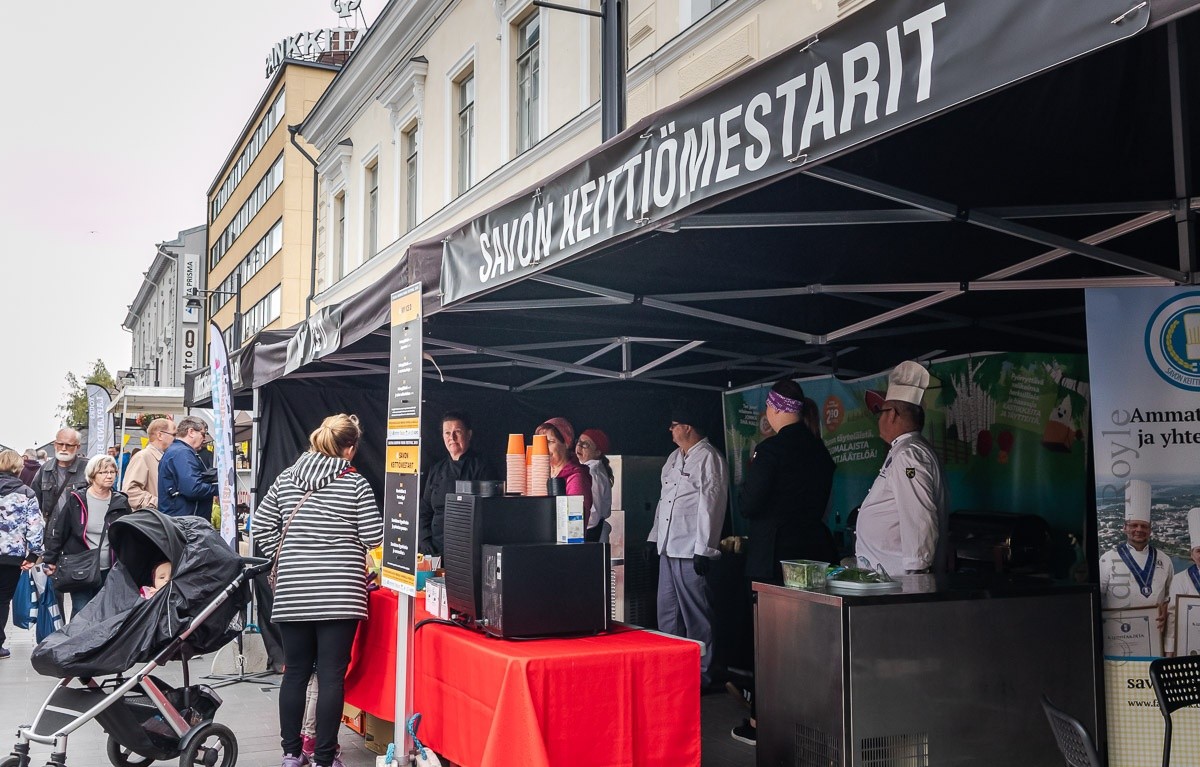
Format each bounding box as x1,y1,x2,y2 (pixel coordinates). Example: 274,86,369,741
418,362,945,748
1099,480,1200,657
252,413,382,767
0,416,218,657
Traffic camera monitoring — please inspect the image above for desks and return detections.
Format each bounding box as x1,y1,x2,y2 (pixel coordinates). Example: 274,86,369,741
341,578,707,767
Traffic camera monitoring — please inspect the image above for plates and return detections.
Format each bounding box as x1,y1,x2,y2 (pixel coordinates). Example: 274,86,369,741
828,579,904,590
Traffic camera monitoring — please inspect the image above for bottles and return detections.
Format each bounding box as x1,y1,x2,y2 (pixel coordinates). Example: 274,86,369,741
237,454,247,469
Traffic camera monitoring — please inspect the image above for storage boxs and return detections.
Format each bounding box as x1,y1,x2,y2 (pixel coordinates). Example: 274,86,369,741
780,559,831,589
365,712,395,754
342,701,366,736
556,495,585,544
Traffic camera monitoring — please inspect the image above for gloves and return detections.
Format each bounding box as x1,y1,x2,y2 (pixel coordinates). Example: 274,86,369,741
692,554,709,577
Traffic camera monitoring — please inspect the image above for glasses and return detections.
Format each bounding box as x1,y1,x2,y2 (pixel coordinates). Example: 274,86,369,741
200,432,206,438
876,408,899,418
55,442,80,449
97,471,118,475
160,430,176,437
667,423,679,431
576,440,595,452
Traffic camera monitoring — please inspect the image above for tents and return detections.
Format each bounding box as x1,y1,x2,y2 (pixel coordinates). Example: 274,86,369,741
250,0,1200,767
182,328,297,417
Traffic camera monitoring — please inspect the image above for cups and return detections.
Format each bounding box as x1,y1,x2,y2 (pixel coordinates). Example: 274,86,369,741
992,544,1010,575
548,477,566,496
417,571,436,591
505,433,551,497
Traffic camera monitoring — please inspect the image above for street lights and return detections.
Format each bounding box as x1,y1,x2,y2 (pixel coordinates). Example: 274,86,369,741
124,357,160,386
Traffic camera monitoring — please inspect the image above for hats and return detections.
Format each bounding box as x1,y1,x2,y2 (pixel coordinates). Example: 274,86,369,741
1124,480,1151,523
885,360,930,405
584,428,608,453
534,417,575,450
1187,507,1200,549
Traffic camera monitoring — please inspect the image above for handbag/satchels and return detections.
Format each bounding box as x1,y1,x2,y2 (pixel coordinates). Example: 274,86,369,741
32,562,47,596
53,547,102,593
12,565,38,630
36,576,63,644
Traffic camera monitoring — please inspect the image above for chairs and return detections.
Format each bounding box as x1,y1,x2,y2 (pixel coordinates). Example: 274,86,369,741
1038,694,1103,767
1149,655,1200,767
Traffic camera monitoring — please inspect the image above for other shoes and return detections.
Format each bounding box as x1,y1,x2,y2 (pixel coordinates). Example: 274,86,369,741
281,752,311,767
310,754,344,767
726,678,755,712
730,721,757,746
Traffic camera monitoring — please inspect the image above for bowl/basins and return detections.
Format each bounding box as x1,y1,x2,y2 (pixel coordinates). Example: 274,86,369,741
779,559,830,588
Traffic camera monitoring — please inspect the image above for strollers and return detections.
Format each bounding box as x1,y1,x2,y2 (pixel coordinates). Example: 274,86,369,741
0,506,275,767
